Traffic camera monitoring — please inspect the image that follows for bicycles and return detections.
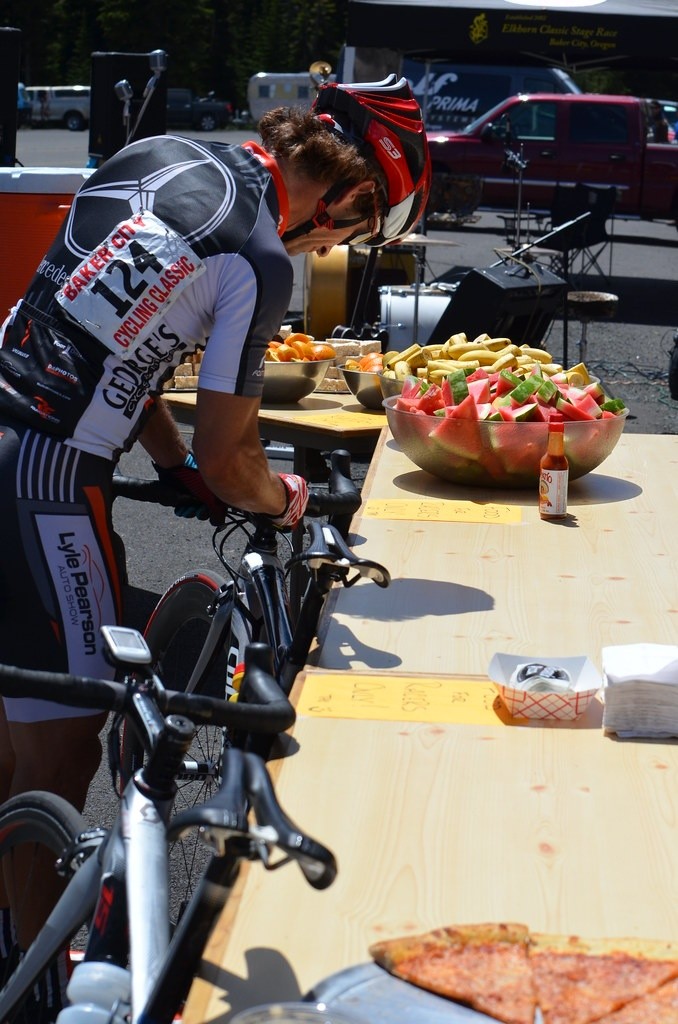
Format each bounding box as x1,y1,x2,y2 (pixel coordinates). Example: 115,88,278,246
106,472,392,939
0,622,337,1021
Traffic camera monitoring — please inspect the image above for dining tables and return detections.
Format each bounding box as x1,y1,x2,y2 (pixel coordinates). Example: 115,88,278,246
179,670,677,1024
155,391,390,482
302,424,678,683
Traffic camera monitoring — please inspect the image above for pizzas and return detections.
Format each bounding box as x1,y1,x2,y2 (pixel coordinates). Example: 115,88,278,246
368,921,678,1024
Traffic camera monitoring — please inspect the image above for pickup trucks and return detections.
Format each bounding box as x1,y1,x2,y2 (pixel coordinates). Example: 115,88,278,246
168,89,233,132
428,94,678,232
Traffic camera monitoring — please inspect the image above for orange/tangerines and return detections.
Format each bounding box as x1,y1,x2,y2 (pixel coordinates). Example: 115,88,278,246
264,332,339,362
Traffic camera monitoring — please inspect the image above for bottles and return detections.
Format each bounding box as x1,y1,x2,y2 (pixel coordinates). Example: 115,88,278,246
538,414,570,520
54,944,131,1022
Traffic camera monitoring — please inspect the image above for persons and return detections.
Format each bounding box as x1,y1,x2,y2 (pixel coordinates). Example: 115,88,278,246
648,98,670,144
16,78,29,132
1,69,433,1024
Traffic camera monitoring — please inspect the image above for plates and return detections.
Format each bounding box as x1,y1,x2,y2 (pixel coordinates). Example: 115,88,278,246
303,963,549,1023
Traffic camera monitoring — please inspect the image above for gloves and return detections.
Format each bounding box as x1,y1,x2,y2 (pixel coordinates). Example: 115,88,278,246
151,450,228,527
267,472,310,533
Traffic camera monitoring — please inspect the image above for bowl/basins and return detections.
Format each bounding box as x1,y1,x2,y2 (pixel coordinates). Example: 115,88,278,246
261,358,333,402
337,364,629,488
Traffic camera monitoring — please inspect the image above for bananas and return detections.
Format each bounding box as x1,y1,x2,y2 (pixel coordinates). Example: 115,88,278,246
382,331,593,387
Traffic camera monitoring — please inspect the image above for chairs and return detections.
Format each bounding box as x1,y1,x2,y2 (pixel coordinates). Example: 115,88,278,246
496,177,622,293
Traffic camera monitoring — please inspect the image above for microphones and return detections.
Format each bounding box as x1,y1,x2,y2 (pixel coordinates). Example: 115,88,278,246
480,122,527,173
143,49,169,97
114,79,133,126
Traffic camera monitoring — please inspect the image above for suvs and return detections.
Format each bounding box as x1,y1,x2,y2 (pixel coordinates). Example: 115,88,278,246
26,86,92,132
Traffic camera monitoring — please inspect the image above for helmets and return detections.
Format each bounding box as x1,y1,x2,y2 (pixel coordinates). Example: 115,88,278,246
307,72,433,248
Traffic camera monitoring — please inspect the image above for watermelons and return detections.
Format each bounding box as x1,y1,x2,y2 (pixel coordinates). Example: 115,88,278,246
390,361,627,485
